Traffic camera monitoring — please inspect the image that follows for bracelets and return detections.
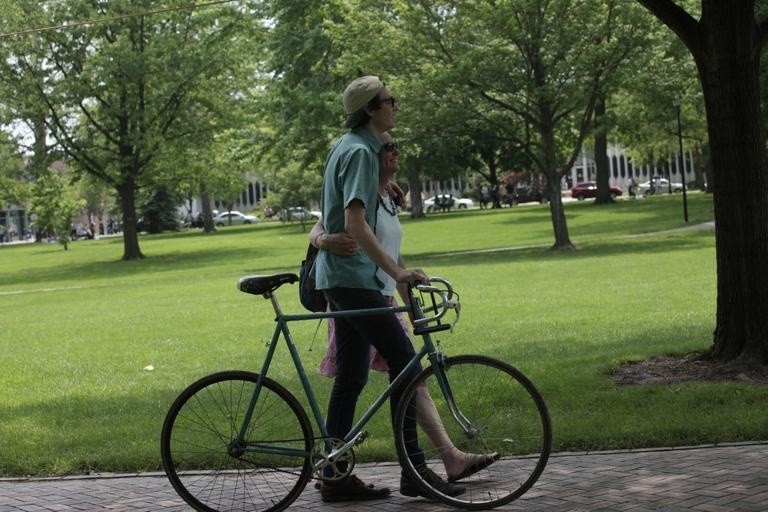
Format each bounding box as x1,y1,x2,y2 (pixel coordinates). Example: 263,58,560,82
313,232,324,249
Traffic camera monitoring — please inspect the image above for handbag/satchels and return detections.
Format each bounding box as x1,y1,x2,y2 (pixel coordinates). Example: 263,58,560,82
296,242,330,313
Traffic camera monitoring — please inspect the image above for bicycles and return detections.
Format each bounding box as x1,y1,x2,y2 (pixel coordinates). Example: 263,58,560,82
158,264,560,510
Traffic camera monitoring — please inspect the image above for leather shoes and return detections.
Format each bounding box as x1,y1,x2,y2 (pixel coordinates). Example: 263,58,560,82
319,476,390,502
400,468,467,500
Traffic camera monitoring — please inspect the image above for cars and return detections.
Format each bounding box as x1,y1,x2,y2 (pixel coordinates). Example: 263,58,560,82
422,193,477,213
110,201,261,233
70,222,94,240
275,207,322,224
635,178,688,197
503,187,553,206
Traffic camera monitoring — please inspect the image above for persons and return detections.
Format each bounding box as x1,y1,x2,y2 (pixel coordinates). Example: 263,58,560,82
307,75,465,504
626,174,662,199
479,179,531,210
432,193,455,213
307,129,502,490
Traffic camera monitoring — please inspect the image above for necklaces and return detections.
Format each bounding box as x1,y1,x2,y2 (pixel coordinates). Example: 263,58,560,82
377,189,396,216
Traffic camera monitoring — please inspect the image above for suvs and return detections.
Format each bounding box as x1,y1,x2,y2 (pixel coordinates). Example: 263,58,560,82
571,180,624,202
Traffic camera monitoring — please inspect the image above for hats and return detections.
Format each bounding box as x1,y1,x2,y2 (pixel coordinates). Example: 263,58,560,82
342,75,383,115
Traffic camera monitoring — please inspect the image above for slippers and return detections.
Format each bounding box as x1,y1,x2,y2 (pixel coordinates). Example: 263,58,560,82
447,451,501,483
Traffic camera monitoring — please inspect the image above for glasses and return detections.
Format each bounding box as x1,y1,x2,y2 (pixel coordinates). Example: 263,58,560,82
379,95,396,106
382,142,394,152
